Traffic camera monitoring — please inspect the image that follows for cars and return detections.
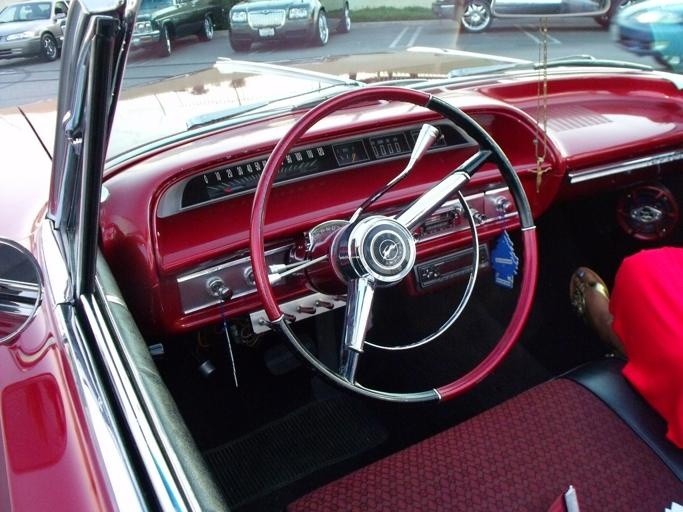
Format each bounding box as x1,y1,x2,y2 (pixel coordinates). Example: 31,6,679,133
434,0,683,75
229,0,350,53
1,1,70,60
130,0,219,57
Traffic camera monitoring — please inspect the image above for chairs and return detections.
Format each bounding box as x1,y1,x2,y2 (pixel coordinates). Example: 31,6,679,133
285,378,683,512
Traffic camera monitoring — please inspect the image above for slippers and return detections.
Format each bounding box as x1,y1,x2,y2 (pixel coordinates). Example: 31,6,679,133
569,267,609,328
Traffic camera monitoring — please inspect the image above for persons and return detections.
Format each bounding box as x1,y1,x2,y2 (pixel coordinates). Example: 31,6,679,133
568,246,683,448
